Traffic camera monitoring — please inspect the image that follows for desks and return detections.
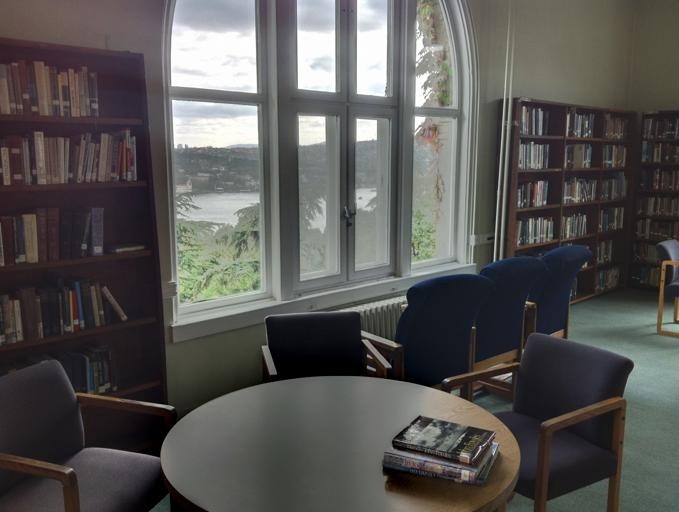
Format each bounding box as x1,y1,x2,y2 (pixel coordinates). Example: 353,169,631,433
159,376,523,511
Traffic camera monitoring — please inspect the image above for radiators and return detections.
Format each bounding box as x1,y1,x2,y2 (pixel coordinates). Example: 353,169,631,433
339,296,409,345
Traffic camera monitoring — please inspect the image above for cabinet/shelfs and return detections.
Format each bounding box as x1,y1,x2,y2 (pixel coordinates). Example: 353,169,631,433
493,96,679,306
1,37,169,458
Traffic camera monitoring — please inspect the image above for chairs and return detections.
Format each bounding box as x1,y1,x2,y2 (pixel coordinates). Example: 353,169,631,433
1,360,176,511
490,332,635,512
540,244,594,340
654,239,679,338
474,254,545,392
259,308,395,383
359,268,481,406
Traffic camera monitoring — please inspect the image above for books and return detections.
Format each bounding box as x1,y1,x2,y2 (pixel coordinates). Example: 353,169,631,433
391,413,494,463
379,448,500,486
1,343,119,400
0,60,100,117
517,105,679,303
382,438,500,482
1,126,137,182
2,207,141,267
0,272,128,345
